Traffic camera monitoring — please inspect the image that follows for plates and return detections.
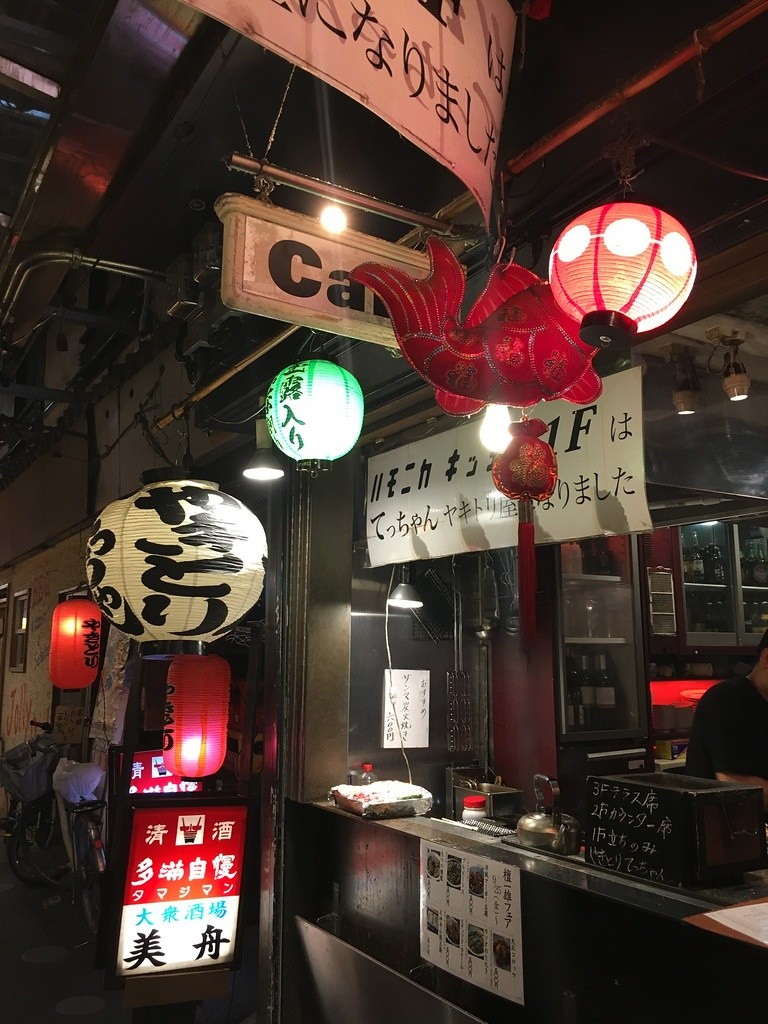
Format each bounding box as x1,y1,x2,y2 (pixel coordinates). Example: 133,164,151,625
468,926,484,956
447,858,461,887
446,917,459,946
494,934,511,972
469,866,484,896
427,852,440,880
427,908,438,930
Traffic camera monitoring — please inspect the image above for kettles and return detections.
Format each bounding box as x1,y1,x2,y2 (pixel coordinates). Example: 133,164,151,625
517,773,581,855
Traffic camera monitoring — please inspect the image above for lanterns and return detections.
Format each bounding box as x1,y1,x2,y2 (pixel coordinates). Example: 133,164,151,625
48,595,101,692
265,351,364,471
548,202,698,352
85,468,268,661
162,654,230,782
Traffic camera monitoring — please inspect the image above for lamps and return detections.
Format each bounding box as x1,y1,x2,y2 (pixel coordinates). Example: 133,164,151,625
242,396,287,482
388,563,424,608
708,334,752,401
668,356,701,414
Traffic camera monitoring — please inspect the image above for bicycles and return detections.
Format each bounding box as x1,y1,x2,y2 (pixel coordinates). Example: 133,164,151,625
6,720,110,946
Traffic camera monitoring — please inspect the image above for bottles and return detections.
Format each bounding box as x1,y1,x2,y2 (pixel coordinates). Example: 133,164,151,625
357,765,378,785
680,531,725,584
462,795,487,821
566,653,618,731
704,601,768,632
739,541,768,587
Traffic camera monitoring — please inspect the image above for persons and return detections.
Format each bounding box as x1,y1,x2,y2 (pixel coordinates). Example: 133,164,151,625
685,627,768,824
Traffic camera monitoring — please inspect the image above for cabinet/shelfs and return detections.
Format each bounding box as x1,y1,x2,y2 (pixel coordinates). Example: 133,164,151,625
554,533,649,743
671,518,768,656
